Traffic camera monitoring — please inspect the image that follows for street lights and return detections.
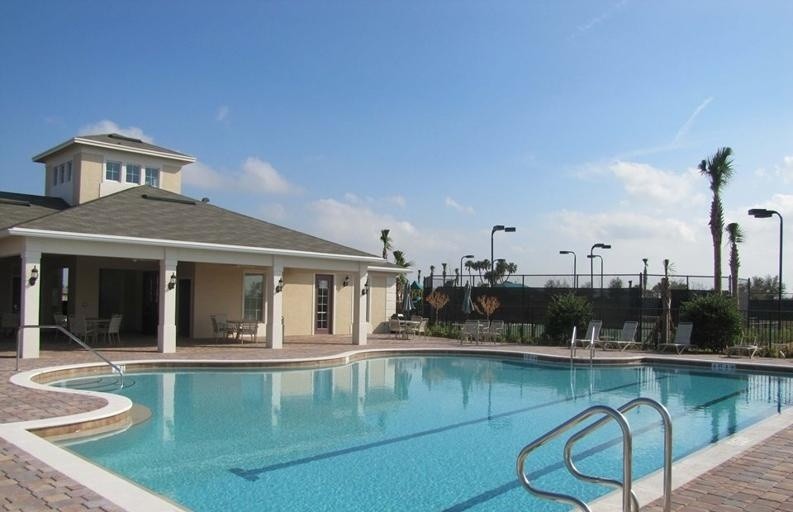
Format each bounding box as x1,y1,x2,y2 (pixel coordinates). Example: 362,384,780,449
589,242,612,289
744,207,783,343
458,250,474,288
488,221,517,288
584,254,604,288
557,249,576,288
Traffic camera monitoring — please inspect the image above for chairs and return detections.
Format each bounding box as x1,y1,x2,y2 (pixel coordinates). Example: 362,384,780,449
451,319,505,346
655,320,697,358
52,308,125,348
210,313,259,345
607,321,642,353
388,314,429,343
569,319,605,352
727,335,763,362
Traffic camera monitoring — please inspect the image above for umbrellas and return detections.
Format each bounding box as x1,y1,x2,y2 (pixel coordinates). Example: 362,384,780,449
403,279,416,319
462,280,475,320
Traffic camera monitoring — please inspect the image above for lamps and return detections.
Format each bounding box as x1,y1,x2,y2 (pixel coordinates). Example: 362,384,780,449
168,271,177,290
275,277,284,292
362,281,369,296
28,265,38,285
